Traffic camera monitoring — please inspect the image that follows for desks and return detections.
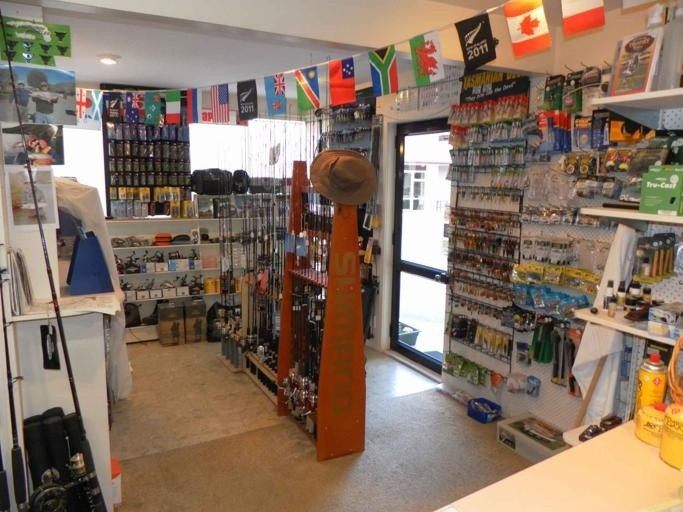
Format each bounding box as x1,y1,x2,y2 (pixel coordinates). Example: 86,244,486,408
14,253,123,512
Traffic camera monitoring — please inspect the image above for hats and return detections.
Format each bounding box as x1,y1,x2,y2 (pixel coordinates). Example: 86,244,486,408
308,149,376,206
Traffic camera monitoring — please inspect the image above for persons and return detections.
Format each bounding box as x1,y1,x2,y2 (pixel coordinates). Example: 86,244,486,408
8,131,38,163
31,81,58,124
9,81,30,122
32,138,59,164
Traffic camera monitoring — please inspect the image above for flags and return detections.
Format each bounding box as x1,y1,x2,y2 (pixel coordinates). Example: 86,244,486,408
236,80,258,119
368,44,398,97
293,66,319,113
210,83,229,124
327,56,356,108
503,0,553,61
454,14,496,74
87,87,201,127
409,30,446,88
263,75,286,116
559,1,604,38
73,89,85,120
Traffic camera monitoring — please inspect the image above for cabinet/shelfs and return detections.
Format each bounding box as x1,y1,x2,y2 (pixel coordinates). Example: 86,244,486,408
573,86,683,352
103,189,289,342
